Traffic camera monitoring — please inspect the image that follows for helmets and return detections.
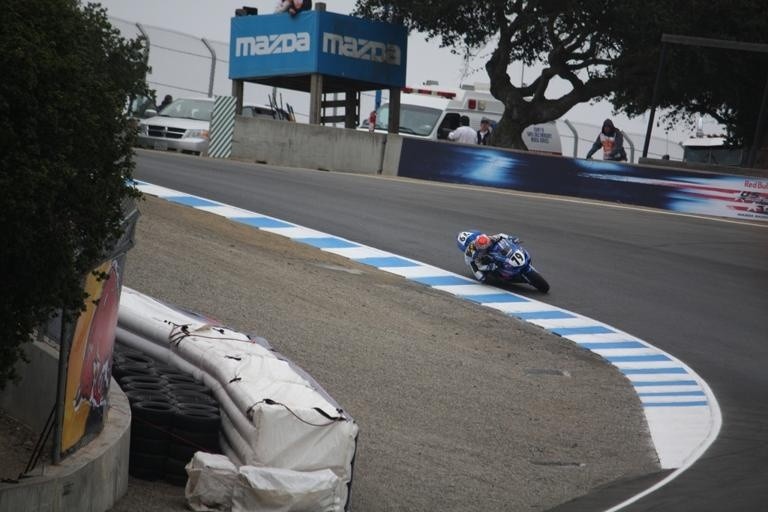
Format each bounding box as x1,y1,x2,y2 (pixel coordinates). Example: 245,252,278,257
474,234,493,253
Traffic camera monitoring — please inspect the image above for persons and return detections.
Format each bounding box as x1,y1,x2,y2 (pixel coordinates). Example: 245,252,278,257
285,1,312,16
273,1,292,14
446,115,479,147
464,231,520,282
586,118,628,162
155,95,173,112
475,117,492,146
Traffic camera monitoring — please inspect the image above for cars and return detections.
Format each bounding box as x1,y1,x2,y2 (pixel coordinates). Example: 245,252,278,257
134,97,294,156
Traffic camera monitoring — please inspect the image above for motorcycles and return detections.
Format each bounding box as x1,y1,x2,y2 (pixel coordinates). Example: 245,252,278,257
455,229,550,294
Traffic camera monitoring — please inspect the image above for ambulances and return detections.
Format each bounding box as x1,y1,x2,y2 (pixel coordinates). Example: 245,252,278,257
356,81,561,155
